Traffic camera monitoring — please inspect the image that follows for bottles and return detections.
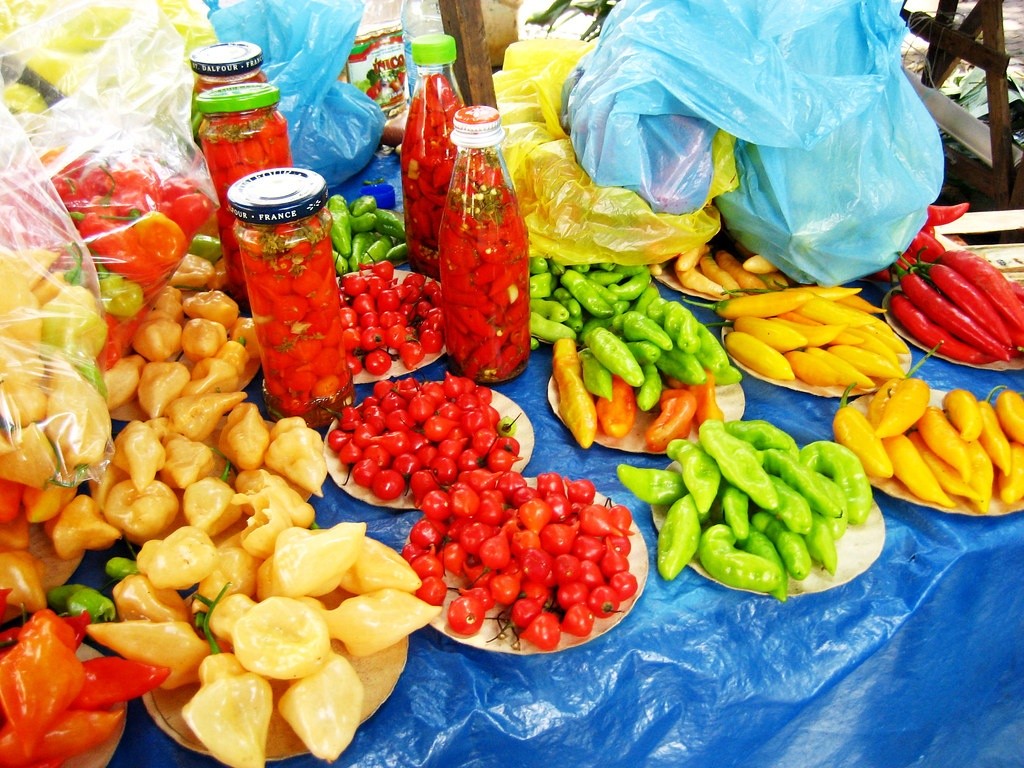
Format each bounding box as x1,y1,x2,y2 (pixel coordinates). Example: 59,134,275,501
196,83,293,314
189,41,268,152
226,167,356,429
400,32,466,279
438,105,530,382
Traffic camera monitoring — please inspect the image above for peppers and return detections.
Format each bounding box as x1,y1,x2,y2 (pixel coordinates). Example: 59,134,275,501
651,202,1024,513
0,0,637,767
524,202,873,602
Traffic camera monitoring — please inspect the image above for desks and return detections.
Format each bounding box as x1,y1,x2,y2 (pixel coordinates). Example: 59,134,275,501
0,261,1023,767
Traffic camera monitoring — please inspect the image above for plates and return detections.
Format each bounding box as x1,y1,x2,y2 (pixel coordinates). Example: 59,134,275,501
882,284,1024,371
335,270,446,384
323,381,534,509
844,389,1024,516
722,318,912,398
406,476,649,654
546,348,746,454
140,590,407,759
55,643,126,767
655,269,722,303
651,459,886,596
